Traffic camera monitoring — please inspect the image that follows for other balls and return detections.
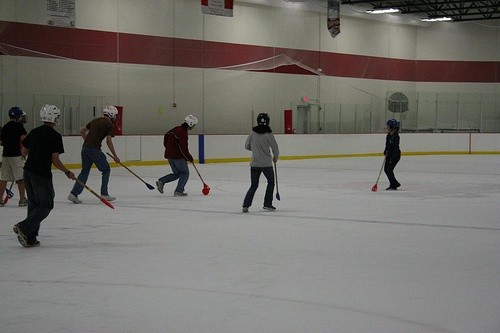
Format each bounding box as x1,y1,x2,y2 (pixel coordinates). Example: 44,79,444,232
202,188,209,195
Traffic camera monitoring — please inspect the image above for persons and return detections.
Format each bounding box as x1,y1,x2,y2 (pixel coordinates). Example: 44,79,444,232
13,104,75,248
242,112,279,213
156,115,198,197
384,118,402,191
67,105,121,204
0,106,27,207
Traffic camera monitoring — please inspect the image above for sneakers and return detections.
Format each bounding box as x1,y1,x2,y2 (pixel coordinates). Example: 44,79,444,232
243,206,249,212
263,205,276,211
19,197,28,206
68,193,81,203
13,224,28,246
26,241,40,247
156,179,164,194
174,191,188,197
101,194,116,202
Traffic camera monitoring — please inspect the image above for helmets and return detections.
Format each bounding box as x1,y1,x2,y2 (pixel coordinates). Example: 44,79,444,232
8,107,22,119
386,119,397,130
103,106,118,119
40,104,61,123
185,114,198,128
22,111,28,123
257,113,270,125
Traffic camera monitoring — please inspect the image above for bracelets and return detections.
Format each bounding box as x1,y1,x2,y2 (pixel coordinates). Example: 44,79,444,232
65,170,71,175
